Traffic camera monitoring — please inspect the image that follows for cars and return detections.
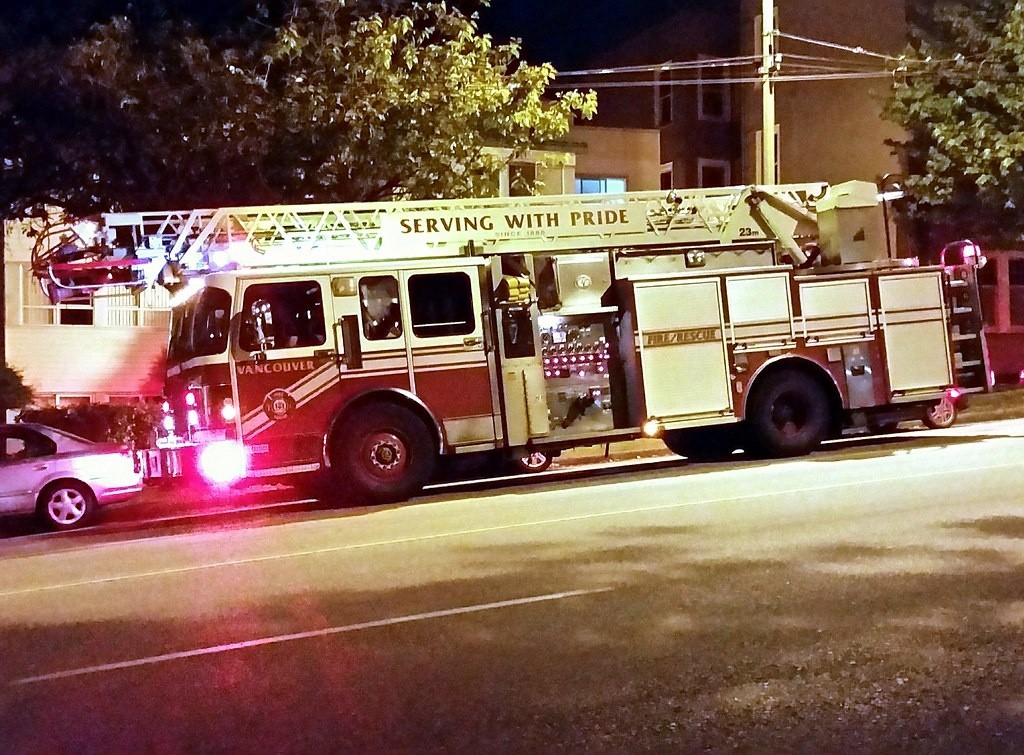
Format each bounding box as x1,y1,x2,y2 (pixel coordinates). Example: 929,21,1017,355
0,424,145,532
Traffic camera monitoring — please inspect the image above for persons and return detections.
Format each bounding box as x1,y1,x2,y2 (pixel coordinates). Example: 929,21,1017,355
276,299,310,347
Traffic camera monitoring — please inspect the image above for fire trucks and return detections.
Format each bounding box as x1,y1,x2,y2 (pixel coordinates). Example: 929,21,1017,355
30,178,994,505
